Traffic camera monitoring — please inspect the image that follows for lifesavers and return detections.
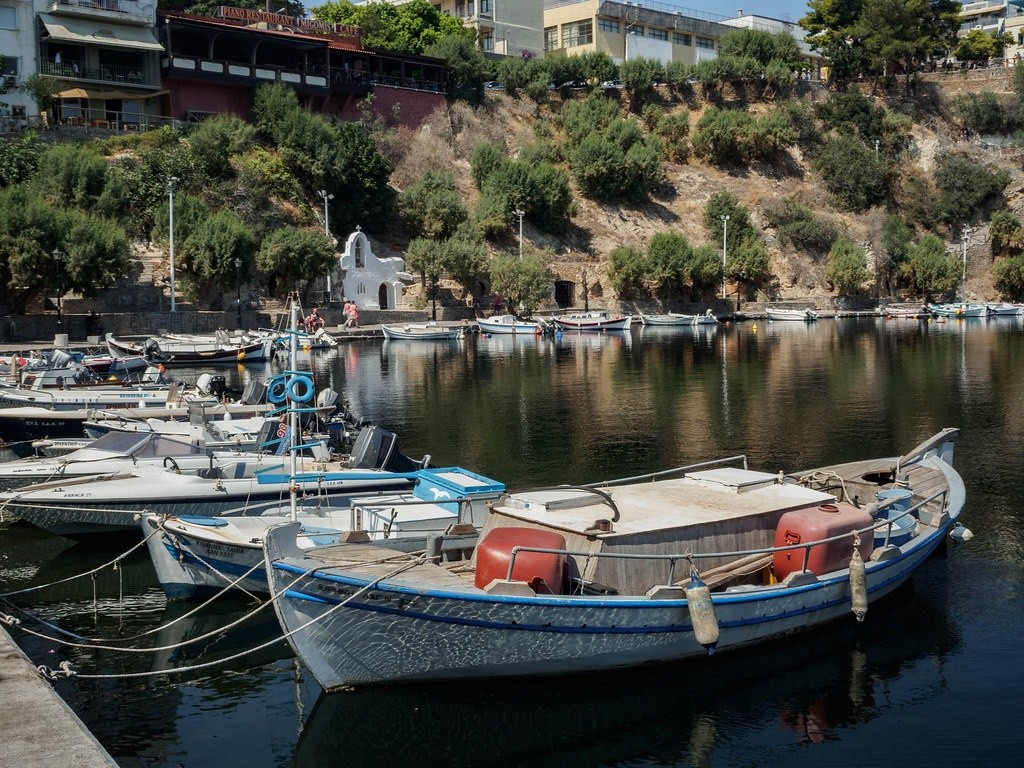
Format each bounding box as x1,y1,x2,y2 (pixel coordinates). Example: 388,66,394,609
266,378,288,404
284,376,315,404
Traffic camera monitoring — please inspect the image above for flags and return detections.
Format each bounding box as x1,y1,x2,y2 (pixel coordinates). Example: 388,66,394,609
996,20,1005,38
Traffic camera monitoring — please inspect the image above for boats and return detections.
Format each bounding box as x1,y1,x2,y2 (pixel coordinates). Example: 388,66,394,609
639,313,717,325
140,467,510,601
766,308,818,321
927,303,1024,317
0,393,434,544
382,322,465,340
0,287,336,459
261,425,969,690
551,312,632,330
878,306,933,319
475,314,539,334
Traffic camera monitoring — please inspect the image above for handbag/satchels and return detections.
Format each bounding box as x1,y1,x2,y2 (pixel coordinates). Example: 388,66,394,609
342,304,346,316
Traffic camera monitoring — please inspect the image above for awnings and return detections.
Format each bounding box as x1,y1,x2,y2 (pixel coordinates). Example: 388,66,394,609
38,13,164,51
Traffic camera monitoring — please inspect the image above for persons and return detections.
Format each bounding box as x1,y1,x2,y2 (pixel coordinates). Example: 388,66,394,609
85,311,104,336
72,61,80,76
899,52,1021,74
465,290,502,318
54,50,62,73
297,300,359,333
794,67,812,80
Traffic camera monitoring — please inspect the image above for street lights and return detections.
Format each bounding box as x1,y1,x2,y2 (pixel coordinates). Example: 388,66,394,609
720,214,730,299
317,190,335,301
167,176,180,311
511,210,525,310
234,257,244,329
961,228,972,301
53,248,65,333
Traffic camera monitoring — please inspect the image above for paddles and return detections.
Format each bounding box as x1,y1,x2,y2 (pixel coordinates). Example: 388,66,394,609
672,546,775,587
706,494,904,589
